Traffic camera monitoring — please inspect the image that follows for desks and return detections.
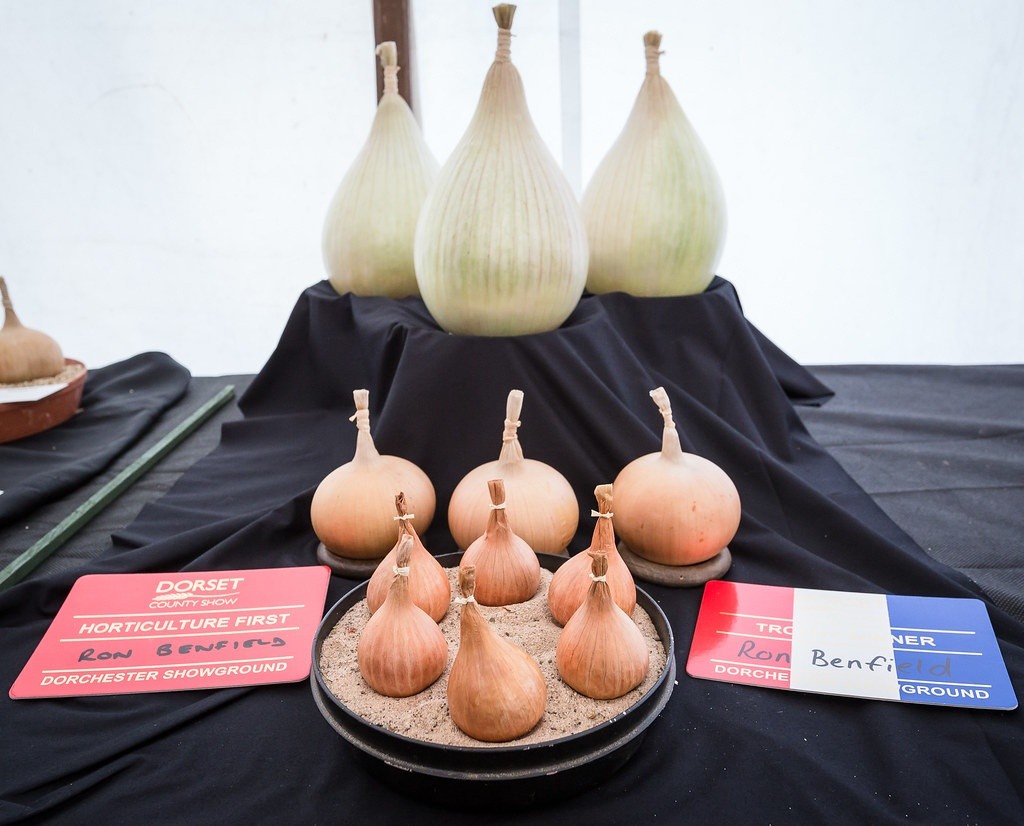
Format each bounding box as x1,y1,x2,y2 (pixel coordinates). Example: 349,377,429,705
0,363,1024,826
